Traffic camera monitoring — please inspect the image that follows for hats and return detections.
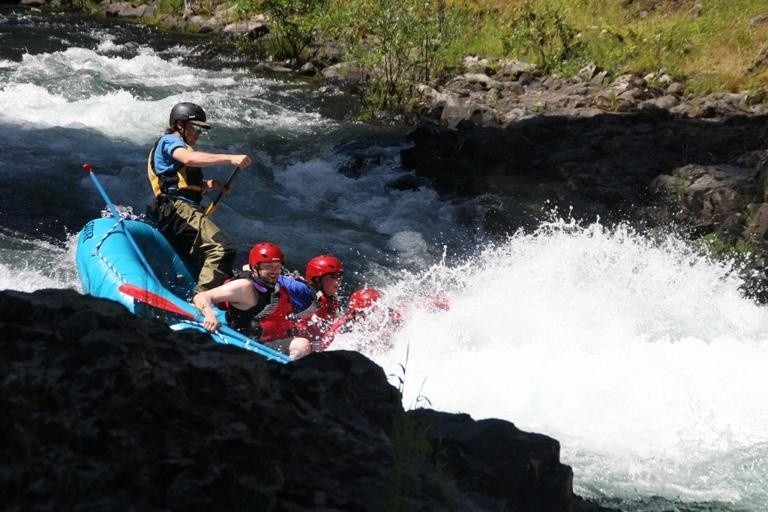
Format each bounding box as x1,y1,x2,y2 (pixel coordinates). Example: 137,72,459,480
188,120,212,131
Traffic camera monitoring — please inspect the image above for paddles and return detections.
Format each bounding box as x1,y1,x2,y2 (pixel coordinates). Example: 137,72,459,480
118,283,297,363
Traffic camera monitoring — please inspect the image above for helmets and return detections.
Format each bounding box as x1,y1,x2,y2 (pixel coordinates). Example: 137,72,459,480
348,289,383,314
248,243,285,272
169,102,206,129
306,254,344,284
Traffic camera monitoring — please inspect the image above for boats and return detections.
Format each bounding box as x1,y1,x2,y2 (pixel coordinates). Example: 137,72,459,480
74,215,302,367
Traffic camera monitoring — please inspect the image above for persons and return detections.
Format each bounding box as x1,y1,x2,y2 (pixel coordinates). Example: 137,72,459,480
192,242,452,363
147,100,252,305
